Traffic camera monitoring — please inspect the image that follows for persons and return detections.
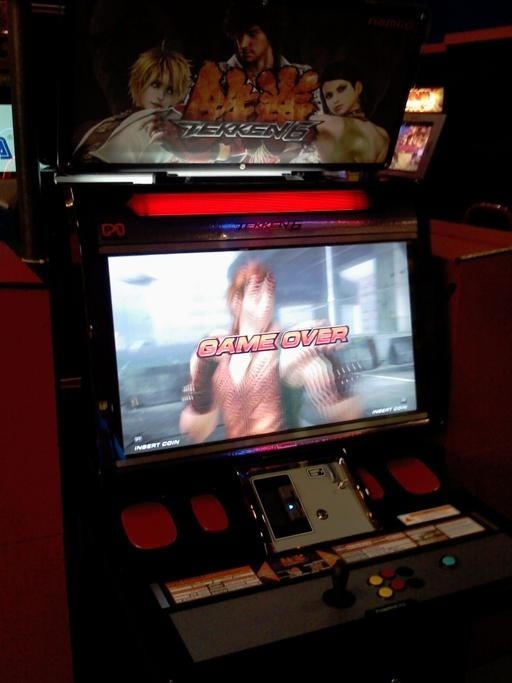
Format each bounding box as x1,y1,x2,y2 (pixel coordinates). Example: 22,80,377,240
174,258,365,440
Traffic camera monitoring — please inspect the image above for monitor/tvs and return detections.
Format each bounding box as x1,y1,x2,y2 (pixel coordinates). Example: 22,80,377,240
387,121,433,172
91,232,429,466
